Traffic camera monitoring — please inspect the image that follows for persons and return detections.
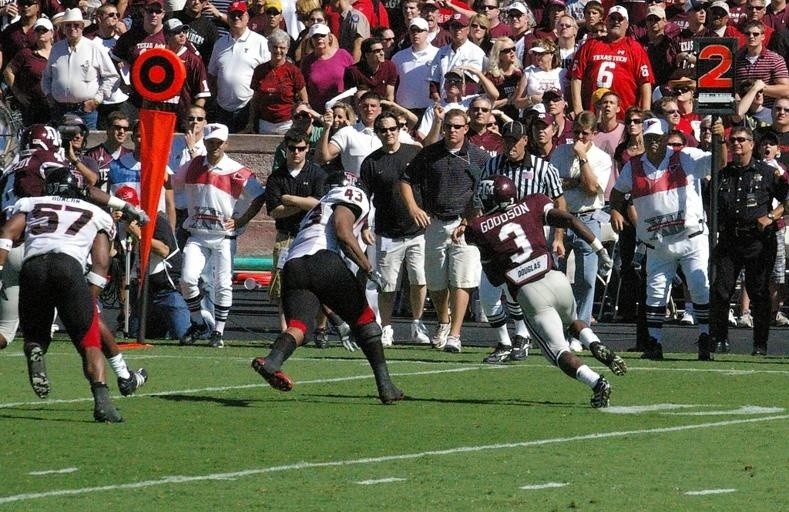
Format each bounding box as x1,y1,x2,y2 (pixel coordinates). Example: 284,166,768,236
397,1,423,50
731,81,774,130
740,1,774,49
466,177,627,408
353,0,389,28
750,98,789,173
273,105,331,170
111,1,166,102
550,112,610,352
399,1,453,51
591,23,608,40
479,0,512,37
266,128,330,349
107,122,176,239
252,173,403,404
0,1,41,70
85,6,127,51
708,127,789,355
541,88,574,146
343,38,396,103
59,115,100,188
527,114,559,162
468,13,492,58
331,102,356,135
575,1,603,43
609,118,725,360
626,1,678,41
591,92,624,159
374,28,395,61
401,110,490,353
4,19,53,126
615,107,646,169
635,6,681,90
112,187,191,342
164,124,265,348
303,25,355,126
737,132,789,329
162,18,211,116
567,6,653,117
693,2,746,48
552,15,580,69
262,1,291,50
384,103,444,147
352,85,370,110
416,66,500,140
85,111,134,192
247,30,308,134
207,2,272,134
731,21,789,100
500,3,533,62
766,1,788,30
0,125,150,397
485,37,522,117
534,0,567,43
514,40,571,122
248,0,284,37
0,168,122,423
330,0,370,65
356,113,431,346
41,8,119,128
667,129,687,149
162,0,219,67
427,13,486,101
315,92,446,187
295,1,320,51
476,121,566,364
167,106,206,252
467,96,503,158
300,9,339,76
652,98,701,148
665,76,700,139
672,1,709,56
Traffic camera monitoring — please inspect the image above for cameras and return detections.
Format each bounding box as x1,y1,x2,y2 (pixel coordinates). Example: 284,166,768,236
46,120,81,143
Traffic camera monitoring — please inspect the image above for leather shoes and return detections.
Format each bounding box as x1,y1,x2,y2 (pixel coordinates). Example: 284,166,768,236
751,345,767,357
712,337,730,353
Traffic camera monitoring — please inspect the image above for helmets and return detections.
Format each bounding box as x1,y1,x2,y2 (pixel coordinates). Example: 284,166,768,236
41,165,89,199
20,123,62,157
472,176,517,218
323,169,370,200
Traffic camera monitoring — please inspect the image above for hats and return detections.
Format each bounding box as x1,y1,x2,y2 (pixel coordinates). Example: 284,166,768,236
527,45,555,54
163,18,190,37
203,123,229,142
62,8,92,28
591,87,610,105
646,6,666,20
144,0,165,7
502,120,528,145
449,13,469,26
422,0,440,8
228,0,247,13
709,1,730,17
608,5,628,20
444,67,464,80
668,77,696,89
408,16,429,29
32,18,53,31
531,112,555,125
304,24,330,41
541,86,562,100
264,0,283,13
643,117,669,135
543,0,565,7
507,1,527,15
684,0,710,13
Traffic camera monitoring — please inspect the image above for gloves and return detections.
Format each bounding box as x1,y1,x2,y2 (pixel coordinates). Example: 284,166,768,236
367,267,388,294
336,318,361,352
598,248,614,277
121,203,151,227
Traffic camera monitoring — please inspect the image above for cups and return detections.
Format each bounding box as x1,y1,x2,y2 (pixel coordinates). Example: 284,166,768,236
244,278,261,290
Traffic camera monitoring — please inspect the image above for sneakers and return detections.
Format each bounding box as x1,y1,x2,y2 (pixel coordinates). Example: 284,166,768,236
737,311,754,329
90,383,124,423
378,384,405,405
569,337,583,353
116,367,149,398
698,332,715,361
663,312,679,322
310,327,329,350
509,336,530,361
409,319,431,345
430,316,452,349
251,357,293,392
480,341,514,364
207,331,224,349
640,339,664,361
381,324,396,348
776,310,789,328
24,341,52,400
589,342,628,377
590,375,613,409
728,309,738,327
444,336,462,353
680,308,699,326
179,318,213,347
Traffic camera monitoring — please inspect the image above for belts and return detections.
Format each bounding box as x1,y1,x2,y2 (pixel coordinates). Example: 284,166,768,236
430,214,462,222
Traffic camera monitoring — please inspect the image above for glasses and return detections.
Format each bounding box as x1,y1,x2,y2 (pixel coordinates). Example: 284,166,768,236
286,144,307,152
18,1,38,7
370,47,385,53
410,28,426,33
559,23,574,28
667,109,683,116
444,123,466,129
35,28,48,33
573,129,591,136
111,124,131,131
747,5,766,11
310,17,323,22
481,5,499,10
624,118,644,125
759,140,776,146
268,45,287,50
772,106,789,113
186,116,205,122
471,106,490,113
379,125,399,132
729,136,751,143
65,23,81,28
471,23,488,30
106,12,120,18
311,33,326,40
743,31,762,36
143,8,164,15
383,36,397,42
508,13,521,17
543,96,560,102
667,142,684,147
499,46,517,54
671,85,689,96
